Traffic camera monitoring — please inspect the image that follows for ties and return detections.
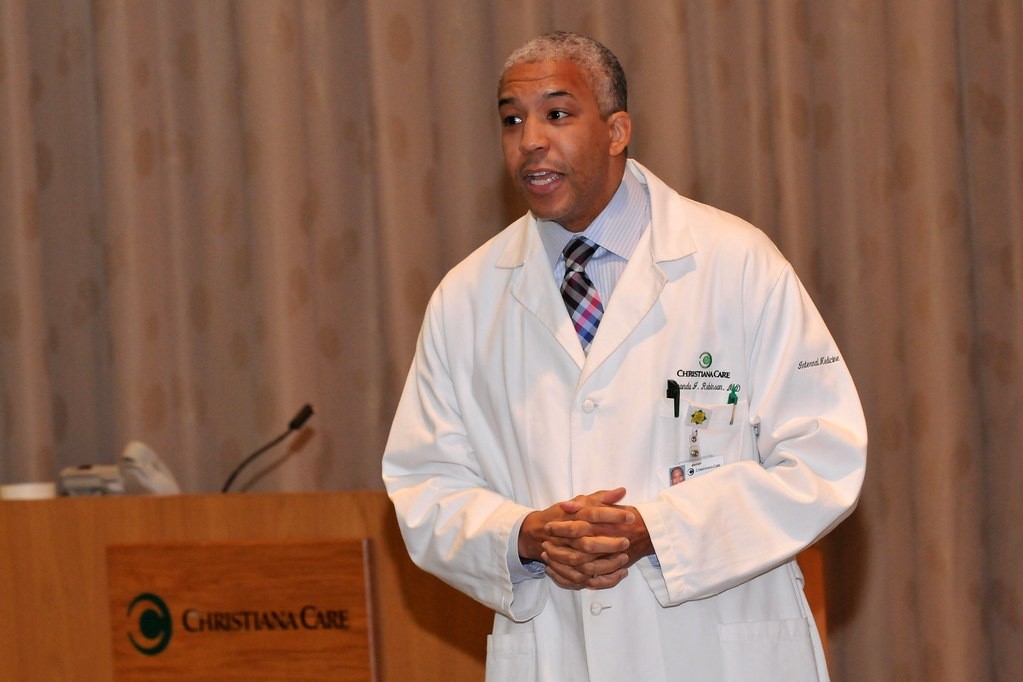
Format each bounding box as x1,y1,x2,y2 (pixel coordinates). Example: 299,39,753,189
559,236,604,360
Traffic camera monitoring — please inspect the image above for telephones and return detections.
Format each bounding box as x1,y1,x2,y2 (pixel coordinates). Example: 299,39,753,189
58,439,179,496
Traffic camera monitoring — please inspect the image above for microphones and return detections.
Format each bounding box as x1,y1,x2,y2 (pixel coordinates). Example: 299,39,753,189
221,404,315,494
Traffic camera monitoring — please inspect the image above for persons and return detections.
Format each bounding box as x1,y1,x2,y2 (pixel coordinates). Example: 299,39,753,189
379,30,867,681
